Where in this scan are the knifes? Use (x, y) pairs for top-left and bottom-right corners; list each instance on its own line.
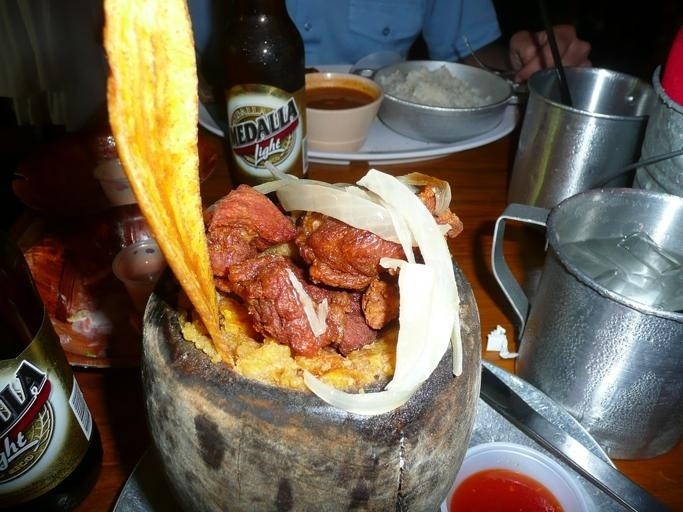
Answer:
(478, 363), (669, 512)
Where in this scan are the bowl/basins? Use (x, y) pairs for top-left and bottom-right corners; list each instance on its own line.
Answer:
(370, 60), (514, 145)
(304, 71), (385, 153)
(437, 440), (597, 512)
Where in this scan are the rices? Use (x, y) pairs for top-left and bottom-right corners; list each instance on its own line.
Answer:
(378, 65), (491, 107)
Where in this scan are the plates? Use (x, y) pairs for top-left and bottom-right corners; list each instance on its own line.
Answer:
(196, 62), (520, 168)
(113, 356), (639, 512)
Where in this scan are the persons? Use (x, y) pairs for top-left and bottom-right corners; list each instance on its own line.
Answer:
(186, 1), (594, 90)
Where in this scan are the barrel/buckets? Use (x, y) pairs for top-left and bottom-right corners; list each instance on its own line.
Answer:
(632, 63), (682, 200)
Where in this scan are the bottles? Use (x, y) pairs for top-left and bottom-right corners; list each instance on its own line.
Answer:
(218, 1), (309, 189)
(0, 227), (104, 512)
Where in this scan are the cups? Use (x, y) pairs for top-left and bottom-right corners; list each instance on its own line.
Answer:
(488, 185), (682, 463)
(504, 64), (655, 209)
